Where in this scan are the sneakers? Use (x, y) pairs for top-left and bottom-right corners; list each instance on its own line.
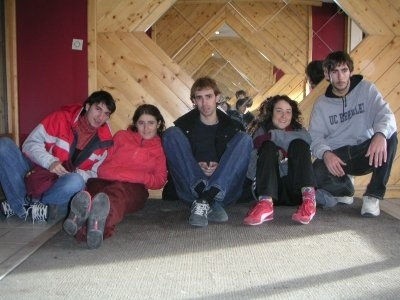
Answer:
(243, 200), (274, 225)
(361, 196), (380, 217)
(23, 197), (59, 224)
(0, 200), (17, 221)
(333, 196), (354, 205)
(189, 200), (210, 226)
(292, 192), (316, 224)
(207, 203), (228, 222)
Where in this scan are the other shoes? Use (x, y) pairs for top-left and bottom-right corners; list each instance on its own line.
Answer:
(63, 191), (91, 236)
(87, 192), (110, 249)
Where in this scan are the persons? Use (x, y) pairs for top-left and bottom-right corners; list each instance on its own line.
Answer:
(308, 51), (399, 217)
(216, 90), (274, 129)
(243, 95), (317, 225)
(306, 60), (324, 89)
(64, 104), (169, 249)
(161, 76), (254, 227)
(1, 90), (116, 225)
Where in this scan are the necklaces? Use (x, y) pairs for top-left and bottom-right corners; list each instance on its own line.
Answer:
(79, 133), (94, 149)
